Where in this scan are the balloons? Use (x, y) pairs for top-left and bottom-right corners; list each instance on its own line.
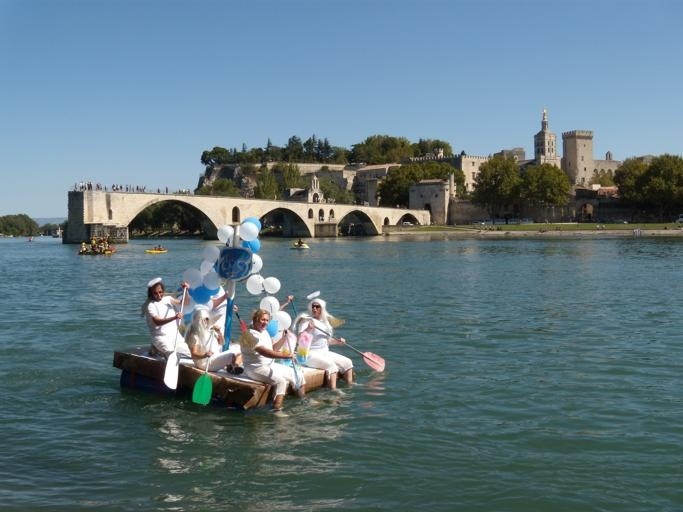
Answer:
(175, 216), (297, 353)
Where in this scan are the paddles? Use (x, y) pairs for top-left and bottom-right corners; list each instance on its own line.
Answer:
(313, 325), (385, 372)
(164, 286), (186, 390)
(235, 312), (246, 334)
(192, 330), (214, 406)
(285, 334), (303, 389)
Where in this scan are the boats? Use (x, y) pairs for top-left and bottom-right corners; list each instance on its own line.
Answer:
(26, 228), (314, 257)
(108, 331), (362, 408)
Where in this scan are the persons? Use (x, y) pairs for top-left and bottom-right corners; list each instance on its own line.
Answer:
(298, 238), (302, 245)
(80, 235), (112, 253)
(296, 298), (352, 388)
(157, 244), (161, 249)
(72, 179), (168, 194)
(181, 303), (242, 374)
(238, 310), (307, 409)
(139, 275), (192, 360)
(211, 285), (239, 355)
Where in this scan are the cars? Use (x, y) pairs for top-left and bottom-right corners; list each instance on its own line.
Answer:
(609, 217), (628, 224)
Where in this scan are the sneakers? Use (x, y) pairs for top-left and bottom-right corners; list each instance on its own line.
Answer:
(227, 366), (243, 375)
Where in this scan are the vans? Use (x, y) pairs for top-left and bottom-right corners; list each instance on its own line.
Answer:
(674, 214), (682, 223)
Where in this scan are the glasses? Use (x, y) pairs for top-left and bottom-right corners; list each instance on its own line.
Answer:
(312, 304), (320, 308)
